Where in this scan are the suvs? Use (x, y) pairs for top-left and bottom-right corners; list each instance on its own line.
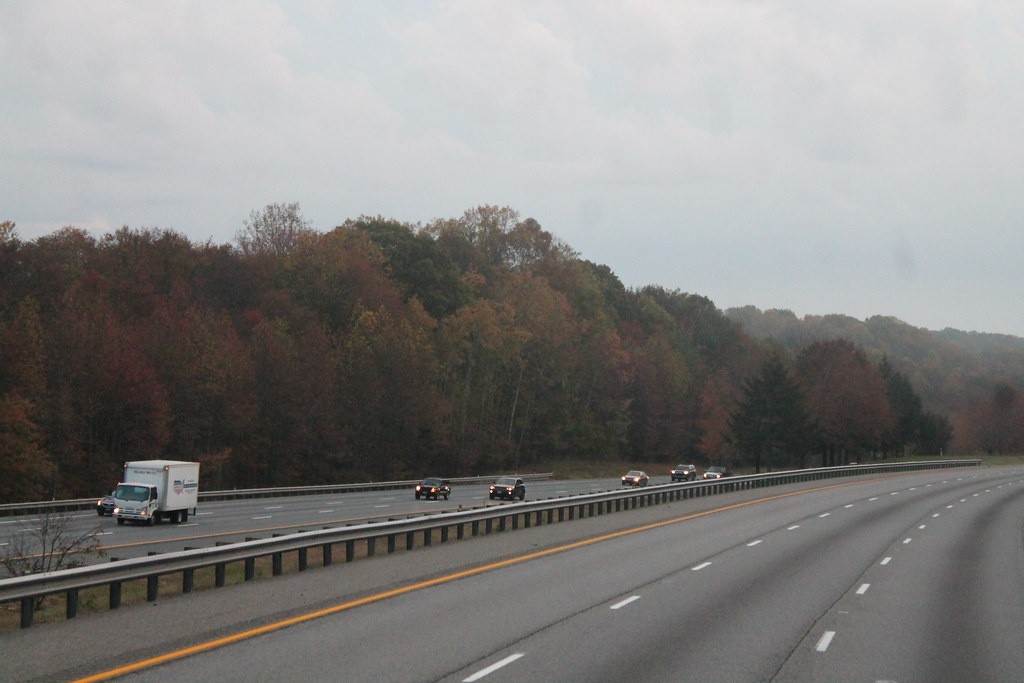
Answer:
(416, 477), (451, 501)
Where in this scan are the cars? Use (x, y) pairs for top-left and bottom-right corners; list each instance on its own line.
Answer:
(97, 494), (118, 517)
(622, 471), (649, 487)
(703, 465), (730, 479)
(490, 477), (525, 500)
(672, 464), (697, 481)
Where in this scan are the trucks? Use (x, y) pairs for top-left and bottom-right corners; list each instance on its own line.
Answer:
(116, 461), (200, 526)
